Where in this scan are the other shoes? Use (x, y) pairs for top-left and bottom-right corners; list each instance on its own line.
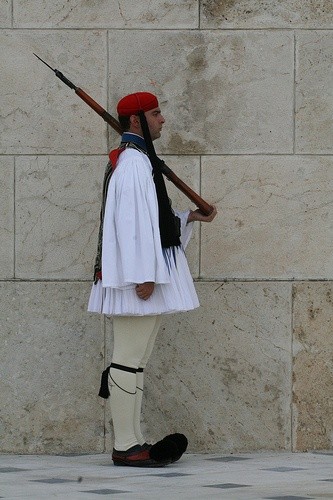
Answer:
(112, 442), (176, 467)
(142, 433), (188, 463)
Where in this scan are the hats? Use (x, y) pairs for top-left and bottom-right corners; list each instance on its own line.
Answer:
(117, 92), (158, 116)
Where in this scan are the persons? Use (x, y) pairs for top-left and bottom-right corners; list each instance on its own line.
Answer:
(87, 91), (217, 466)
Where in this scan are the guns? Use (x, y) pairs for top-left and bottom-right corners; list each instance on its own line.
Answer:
(32, 53), (214, 217)
